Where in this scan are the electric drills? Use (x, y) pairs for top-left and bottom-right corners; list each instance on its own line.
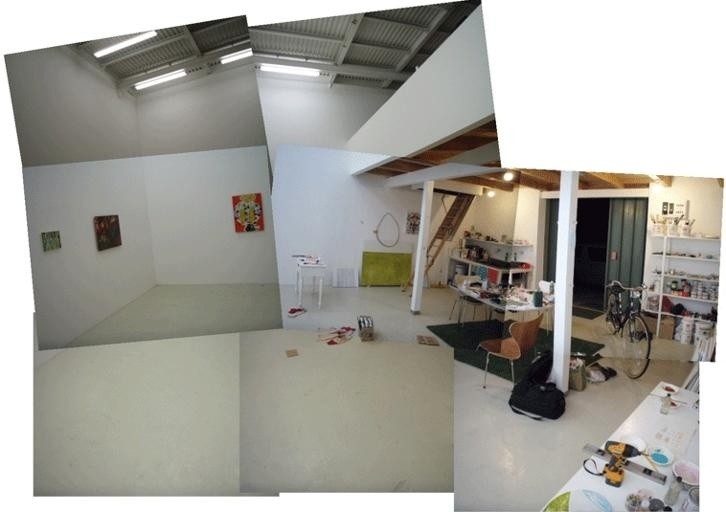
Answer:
(602, 441), (647, 473)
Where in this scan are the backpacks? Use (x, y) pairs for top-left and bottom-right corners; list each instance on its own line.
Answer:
(525, 349), (553, 386)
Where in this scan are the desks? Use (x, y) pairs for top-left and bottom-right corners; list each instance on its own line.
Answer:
(447, 281), (553, 338)
(293, 254), (326, 307)
(540, 381), (698, 512)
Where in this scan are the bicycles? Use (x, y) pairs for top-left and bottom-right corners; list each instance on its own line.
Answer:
(605, 281), (652, 380)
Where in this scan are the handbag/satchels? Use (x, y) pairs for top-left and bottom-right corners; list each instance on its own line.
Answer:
(508, 377), (567, 421)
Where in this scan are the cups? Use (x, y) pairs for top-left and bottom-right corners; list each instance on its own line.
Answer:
(483, 254), (488, 262)
(679, 486), (699, 511)
(651, 223), (695, 239)
(533, 292), (543, 307)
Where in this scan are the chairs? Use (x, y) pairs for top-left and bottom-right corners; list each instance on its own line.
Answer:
(475, 313), (544, 393)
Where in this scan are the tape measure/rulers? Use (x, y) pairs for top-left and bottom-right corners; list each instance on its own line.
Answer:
(603, 463), (625, 487)
(581, 440), (670, 486)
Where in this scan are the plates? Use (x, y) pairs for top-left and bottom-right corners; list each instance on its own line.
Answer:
(670, 399), (680, 409)
(541, 488), (618, 512)
(672, 459), (699, 487)
(620, 434), (646, 453)
(658, 382), (679, 394)
(646, 445), (673, 466)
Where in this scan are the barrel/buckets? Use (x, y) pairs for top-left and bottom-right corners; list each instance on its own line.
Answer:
(681, 318), (694, 332)
(680, 332), (693, 346)
(693, 334), (709, 347)
(694, 320), (712, 335)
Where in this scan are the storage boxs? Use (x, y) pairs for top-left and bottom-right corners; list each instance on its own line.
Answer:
(643, 312), (674, 338)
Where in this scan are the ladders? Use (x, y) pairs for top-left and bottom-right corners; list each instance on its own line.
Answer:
(402, 194), (469, 296)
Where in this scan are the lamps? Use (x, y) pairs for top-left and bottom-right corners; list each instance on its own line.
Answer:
(256, 62), (322, 77)
(219, 46), (253, 65)
(93, 30), (157, 58)
(132, 67), (188, 89)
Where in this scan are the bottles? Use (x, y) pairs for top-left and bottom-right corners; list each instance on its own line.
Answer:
(359, 317), (373, 330)
(664, 475), (682, 506)
(471, 248), (476, 257)
(663, 202), (668, 214)
(670, 279), (719, 302)
(669, 203), (674, 214)
(548, 280), (555, 294)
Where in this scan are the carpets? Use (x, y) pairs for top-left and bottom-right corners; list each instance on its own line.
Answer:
(425, 319), (605, 384)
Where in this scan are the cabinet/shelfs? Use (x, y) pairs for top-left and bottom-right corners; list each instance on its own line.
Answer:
(446, 253), (532, 296)
(644, 233), (721, 324)
